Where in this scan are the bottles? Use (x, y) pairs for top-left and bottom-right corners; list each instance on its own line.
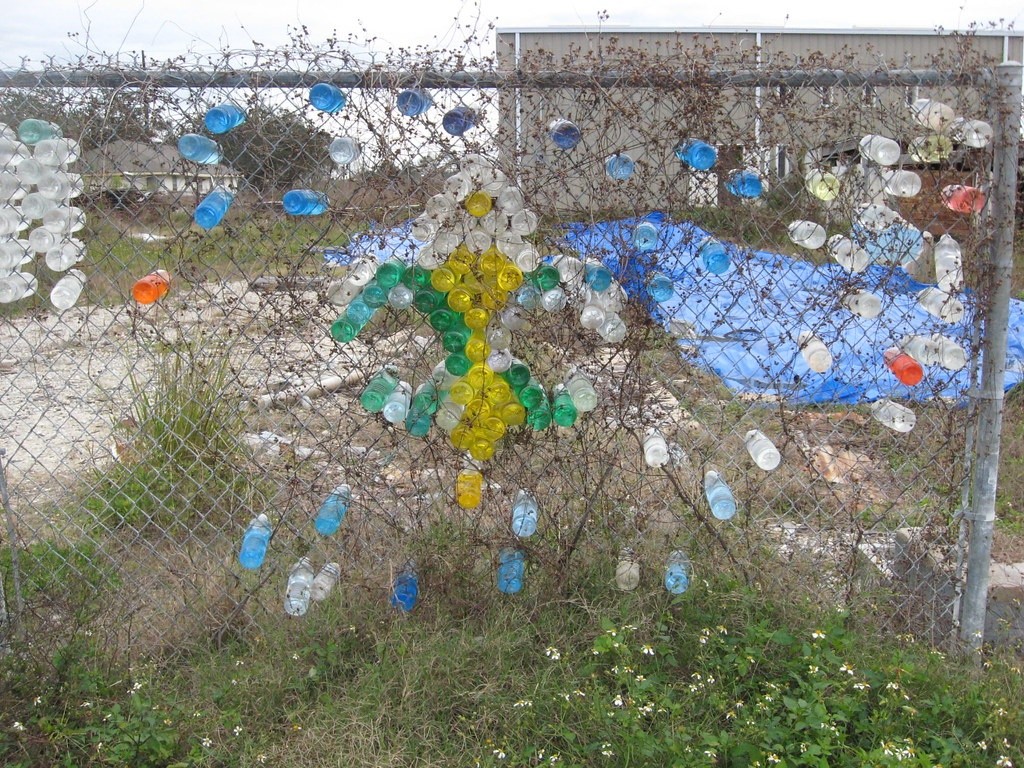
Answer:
(677, 136), (717, 171)
(238, 514), (273, 567)
(195, 184), (233, 228)
(132, 269), (170, 304)
(310, 562), (341, 600)
(664, 550), (692, 594)
(700, 235), (730, 273)
(177, 133), (226, 164)
(644, 411), (669, 469)
(617, 548), (640, 591)
(393, 556), (421, 612)
(205, 102), (247, 134)
(288, 556), (313, 615)
(646, 274), (674, 303)
(274, 83), (632, 537)
(723, 167), (763, 199)
(745, 429), (780, 471)
(499, 548), (525, 594)
(0, 119), (89, 311)
(634, 223), (660, 247)
(703, 470), (736, 520)
(787, 96), (993, 431)
